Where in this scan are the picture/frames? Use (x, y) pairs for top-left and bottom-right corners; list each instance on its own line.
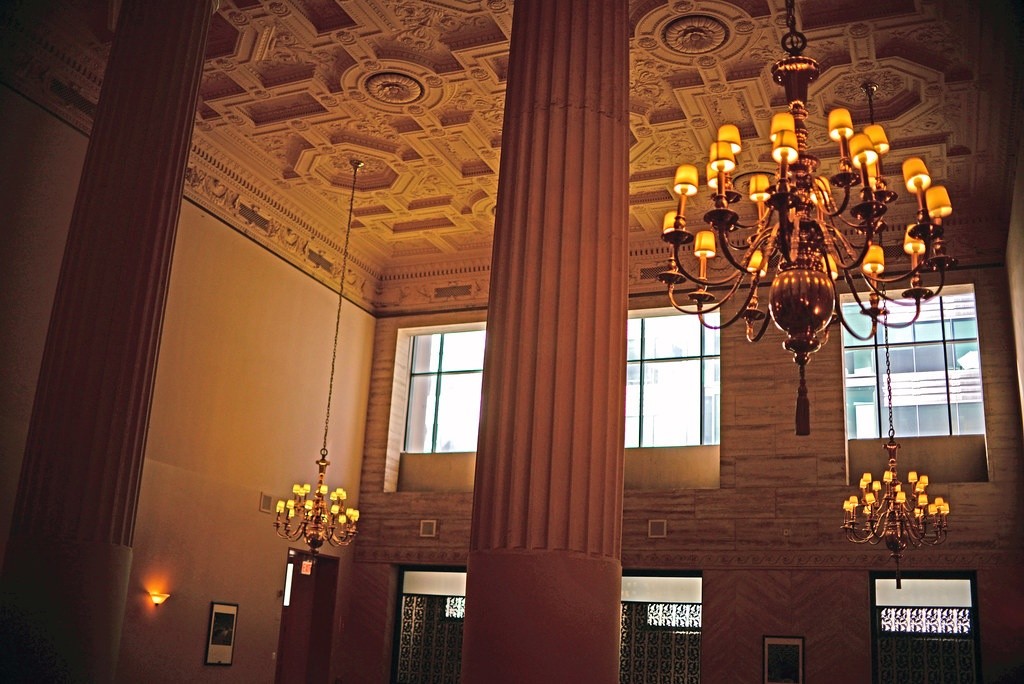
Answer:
(204, 601), (239, 669)
(761, 636), (806, 684)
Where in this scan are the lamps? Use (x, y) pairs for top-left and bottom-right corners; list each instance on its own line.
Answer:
(834, 83), (950, 596)
(148, 592), (170, 607)
(653, 0), (957, 436)
(272, 159), (365, 571)
